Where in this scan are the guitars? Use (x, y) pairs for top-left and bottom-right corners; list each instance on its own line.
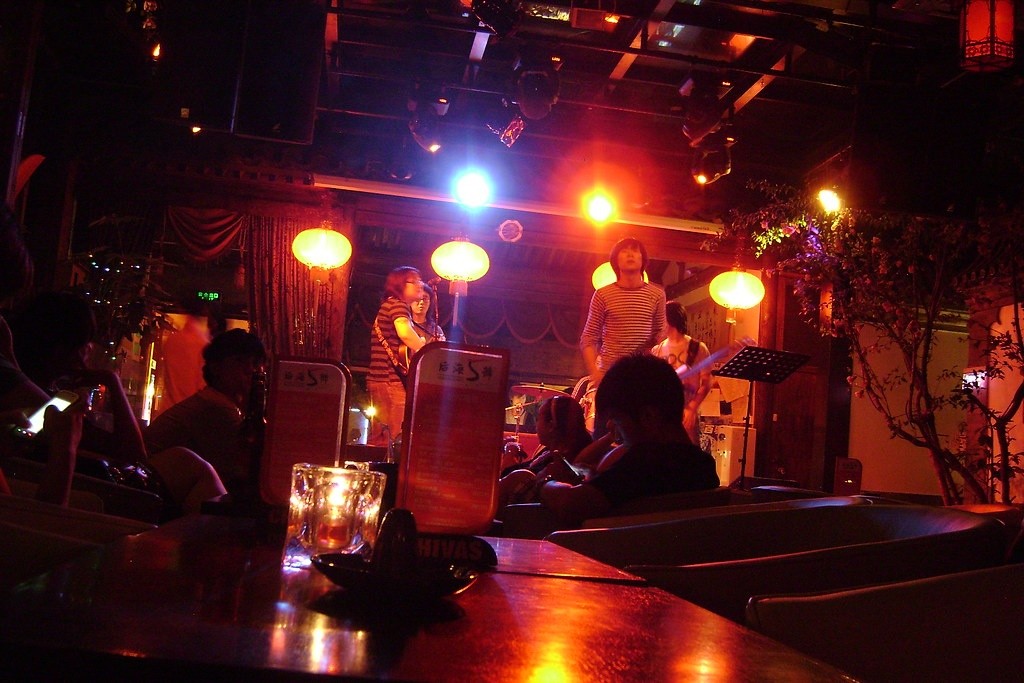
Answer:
(398, 333), (446, 376)
(678, 335), (758, 380)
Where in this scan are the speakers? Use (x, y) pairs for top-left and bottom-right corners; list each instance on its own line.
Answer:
(166, 0), (328, 145)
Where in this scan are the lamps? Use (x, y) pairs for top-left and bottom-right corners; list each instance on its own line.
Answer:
(690, 130), (736, 184)
(408, 119), (441, 151)
(434, 82), (449, 115)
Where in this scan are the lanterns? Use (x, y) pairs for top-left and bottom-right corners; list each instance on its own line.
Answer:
(591, 261), (650, 289)
(292, 219), (353, 318)
(962, 0), (1015, 76)
(708, 264), (766, 325)
(430, 231), (490, 327)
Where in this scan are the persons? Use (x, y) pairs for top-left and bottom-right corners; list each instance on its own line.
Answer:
(501, 396), (593, 512)
(543, 353), (720, 522)
(652, 302), (713, 447)
(143, 328), (262, 479)
(366, 263), (450, 449)
(578, 238), (666, 389)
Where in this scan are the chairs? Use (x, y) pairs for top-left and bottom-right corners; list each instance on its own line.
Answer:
(508, 474), (1024, 683)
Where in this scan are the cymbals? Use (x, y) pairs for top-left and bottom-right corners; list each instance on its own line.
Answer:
(511, 385), (572, 400)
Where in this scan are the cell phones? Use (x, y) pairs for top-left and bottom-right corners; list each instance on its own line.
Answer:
(14, 390), (81, 440)
(560, 456), (582, 479)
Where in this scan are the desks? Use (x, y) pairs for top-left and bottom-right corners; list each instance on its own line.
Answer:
(0, 508), (866, 683)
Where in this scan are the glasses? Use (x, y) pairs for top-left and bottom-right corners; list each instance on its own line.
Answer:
(401, 279), (423, 288)
(414, 297), (432, 304)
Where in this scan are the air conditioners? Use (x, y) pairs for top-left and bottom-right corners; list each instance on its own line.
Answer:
(486, 105), (524, 147)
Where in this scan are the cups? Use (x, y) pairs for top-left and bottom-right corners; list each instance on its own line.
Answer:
(280, 463), (385, 575)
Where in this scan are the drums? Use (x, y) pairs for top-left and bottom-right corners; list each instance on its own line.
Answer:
(579, 387), (598, 435)
